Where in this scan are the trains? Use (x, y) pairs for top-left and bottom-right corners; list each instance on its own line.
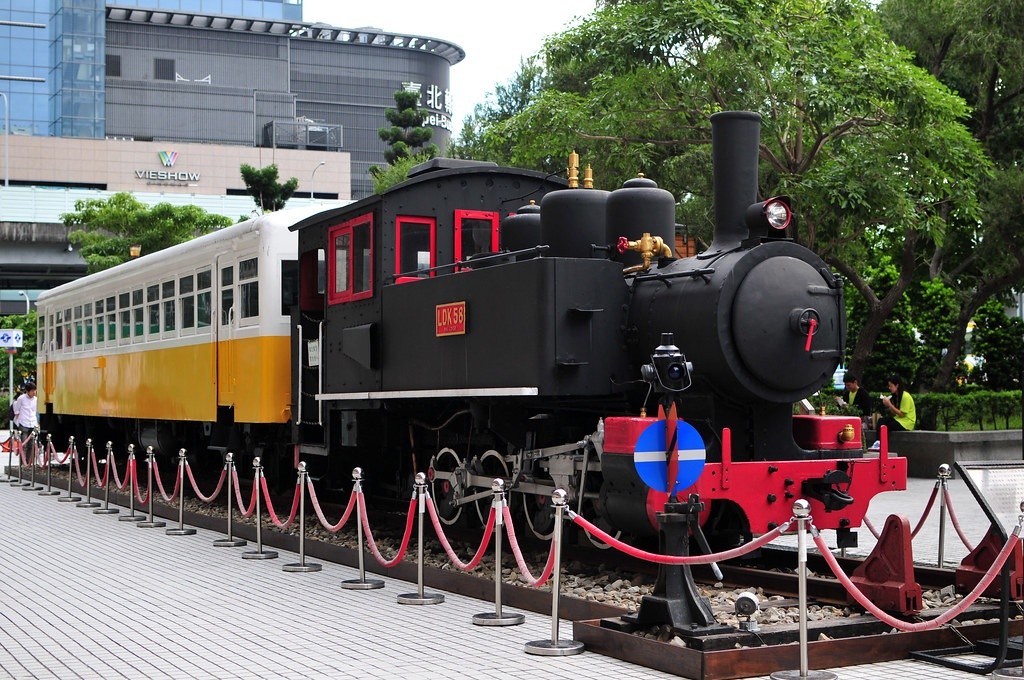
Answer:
(37, 110), (908, 570)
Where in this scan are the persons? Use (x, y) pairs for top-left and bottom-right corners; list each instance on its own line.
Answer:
(9, 392), (24, 431)
(14, 382), (37, 469)
(843, 374), (870, 416)
(868, 375), (916, 450)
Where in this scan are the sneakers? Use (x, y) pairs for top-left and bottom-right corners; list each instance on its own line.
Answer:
(868, 440), (880, 450)
